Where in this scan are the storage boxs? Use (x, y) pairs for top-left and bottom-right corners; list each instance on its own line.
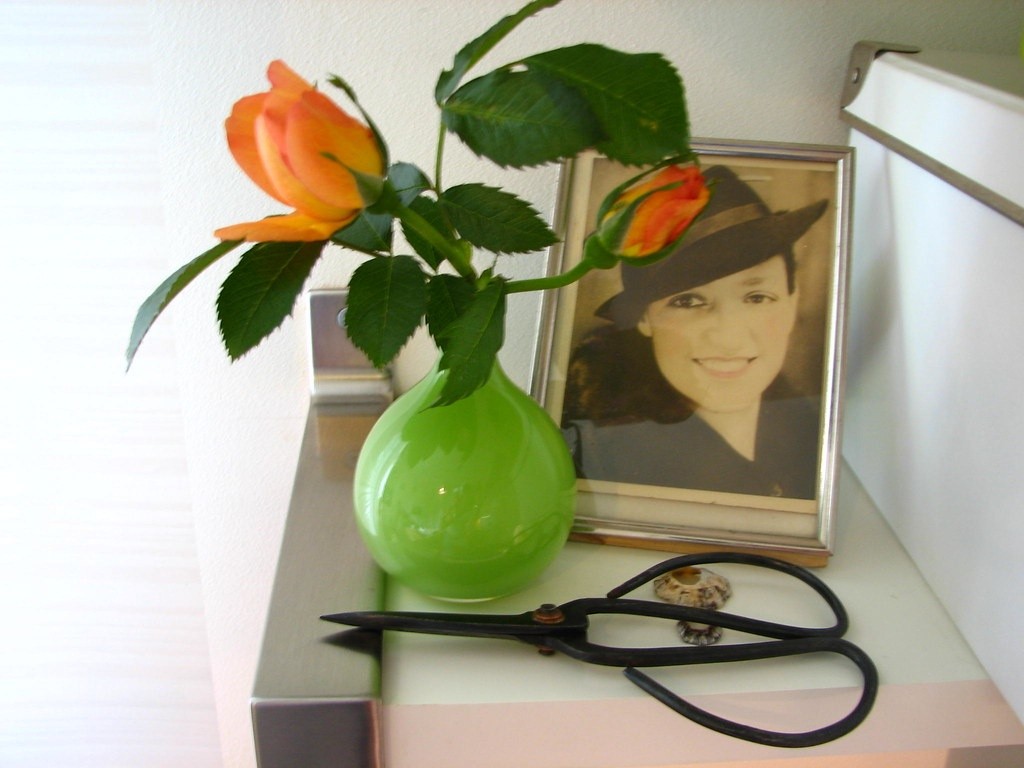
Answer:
(840, 38), (1024, 726)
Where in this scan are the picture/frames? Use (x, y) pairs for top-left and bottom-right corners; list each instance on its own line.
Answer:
(530, 137), (856, 570)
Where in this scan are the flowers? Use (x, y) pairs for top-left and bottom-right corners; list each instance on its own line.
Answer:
(124, 0), (691, 417)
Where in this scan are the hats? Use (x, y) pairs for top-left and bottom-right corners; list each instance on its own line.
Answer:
(593, 164), (829, 329)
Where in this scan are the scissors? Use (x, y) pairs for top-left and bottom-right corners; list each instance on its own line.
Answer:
(321, 550), (878, 748)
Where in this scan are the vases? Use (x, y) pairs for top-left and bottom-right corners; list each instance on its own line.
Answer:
(351, 343), (577, 603)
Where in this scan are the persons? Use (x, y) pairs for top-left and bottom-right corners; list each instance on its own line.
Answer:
(560, 167), (829, 501)
